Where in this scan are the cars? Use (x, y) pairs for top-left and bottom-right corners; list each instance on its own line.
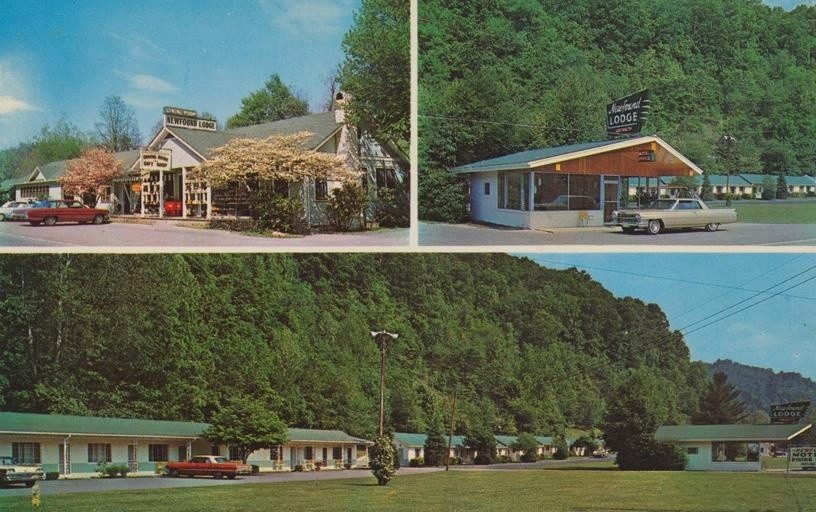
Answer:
(534, 191), (613, 211)
(613, 197), (739, 233)
(592, 447), (610, 458)
(0, 455), (46, 489)
(165, 454), (255, 480)
(0, 200), (40, 222)
(11, 198), (111, 226)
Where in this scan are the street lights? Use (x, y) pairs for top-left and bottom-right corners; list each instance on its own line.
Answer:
(371, 328), (401, 440)
